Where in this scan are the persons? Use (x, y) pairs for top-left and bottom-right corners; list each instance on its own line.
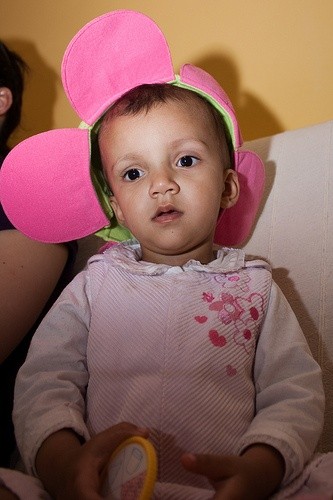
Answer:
(0, 39), (78, 467)
(0, 8), (333, 499)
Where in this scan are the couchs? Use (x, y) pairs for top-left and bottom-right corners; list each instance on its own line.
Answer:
(66, 120), (333, 454)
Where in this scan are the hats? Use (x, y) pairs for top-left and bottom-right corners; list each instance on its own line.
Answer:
(1, 10), (266, 254)
